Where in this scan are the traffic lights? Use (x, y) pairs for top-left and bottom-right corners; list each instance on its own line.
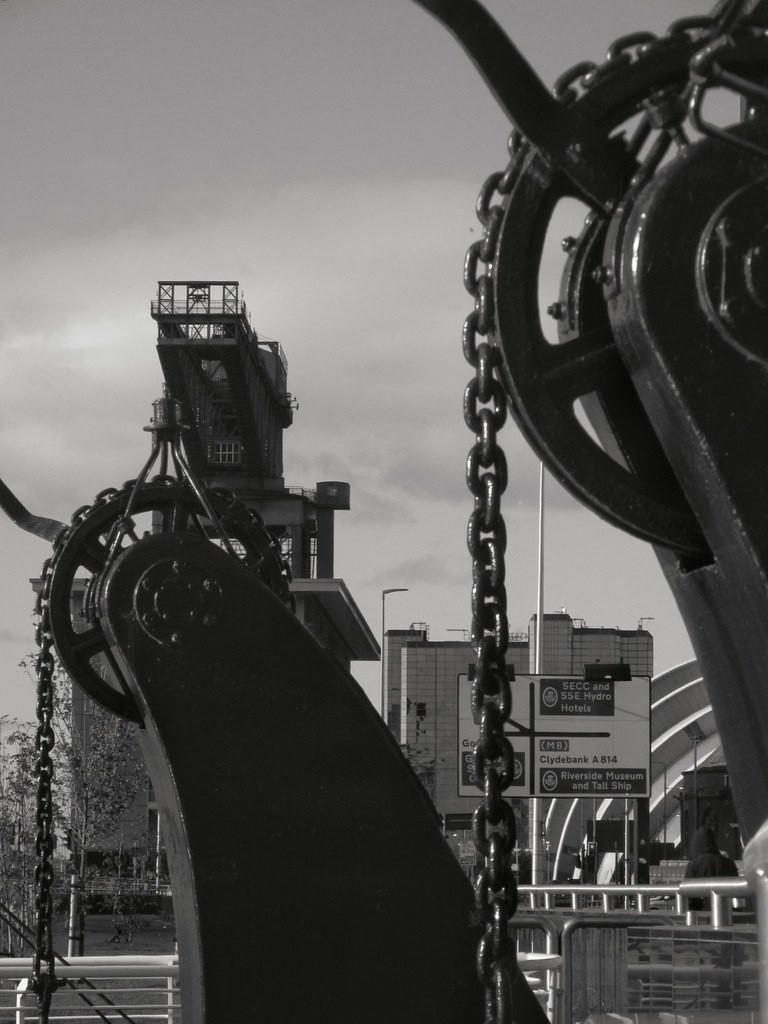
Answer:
(380, 589), (410, 720)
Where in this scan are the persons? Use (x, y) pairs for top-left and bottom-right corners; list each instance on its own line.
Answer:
(685, 826), (738, 911)
